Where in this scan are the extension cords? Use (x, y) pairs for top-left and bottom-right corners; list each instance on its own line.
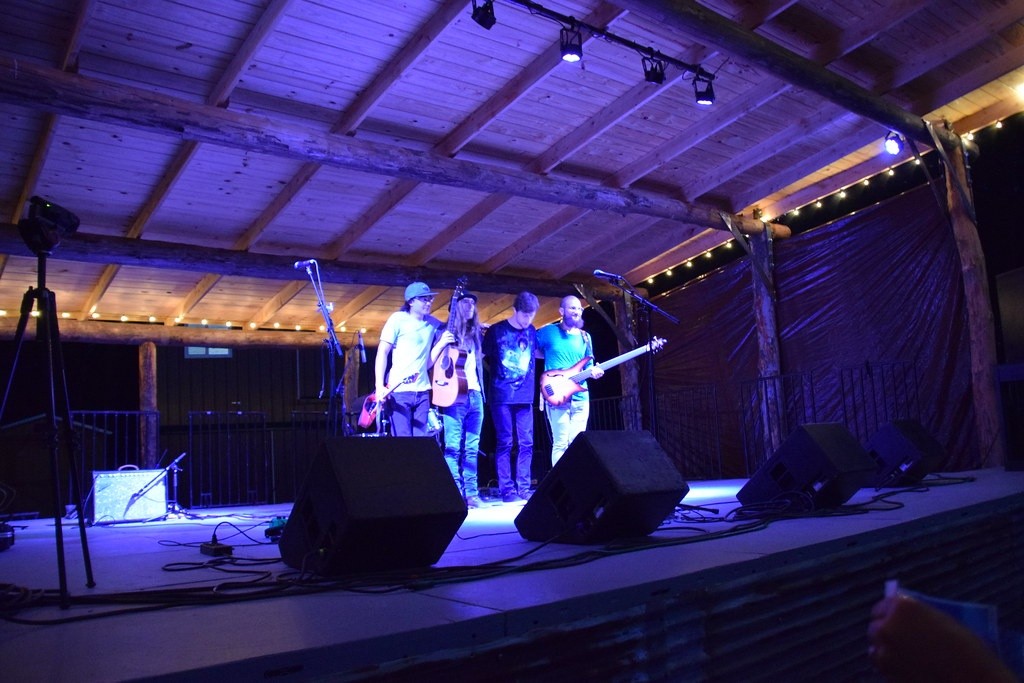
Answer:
(201, 542), (235, 557)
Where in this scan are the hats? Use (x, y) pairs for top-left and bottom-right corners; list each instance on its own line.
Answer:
(447, 290), (477, 313)
(404, 281), (438, 302)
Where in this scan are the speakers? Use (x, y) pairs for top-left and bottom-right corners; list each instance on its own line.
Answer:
(736, 423), (876, 519)
(91, 469), (168, 524)
(515, 429), (691, 544)
(863, 418), (945, 489)
(279, 435), (468, 578)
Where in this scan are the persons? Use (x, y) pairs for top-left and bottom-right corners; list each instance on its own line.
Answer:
(483, 295), (605, 469)
(426, 290), (487, 509)
(867, 595), (1022, 683)
(374, 282), (490, 437)
(481, 291), (545, 503)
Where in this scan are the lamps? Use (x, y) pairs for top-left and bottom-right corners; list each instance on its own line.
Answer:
(692, 68), (715, 105)
(471, 0), (496, 30)
(885, 131), (903, 155)
(641, 47), (666, 84)
(560, 16), (583, 62)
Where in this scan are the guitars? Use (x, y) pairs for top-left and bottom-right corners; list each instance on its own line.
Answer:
(427, 275), (468, 408)
(540, 336), (667, 406)
(358, 372), (420, 429)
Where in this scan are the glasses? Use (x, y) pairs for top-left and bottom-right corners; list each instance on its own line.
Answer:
(414, 296), (435, 303)
(563, 306), (585, 312)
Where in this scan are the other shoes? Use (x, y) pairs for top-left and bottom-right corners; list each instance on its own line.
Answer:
(465, 496), (491, 510)
(502, 489), (524, 505)
(519, 488), (533, 500)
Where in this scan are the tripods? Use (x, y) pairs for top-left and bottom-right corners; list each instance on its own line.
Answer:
(138, 454), (204, 522)
(0, 253), (98, 609)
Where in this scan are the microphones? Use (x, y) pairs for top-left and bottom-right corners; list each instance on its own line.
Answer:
(132, 492), (139, 497)
(593, 269), (624, 280)
(358, 332), (366, 362)
(294, 259), (315, 269)
